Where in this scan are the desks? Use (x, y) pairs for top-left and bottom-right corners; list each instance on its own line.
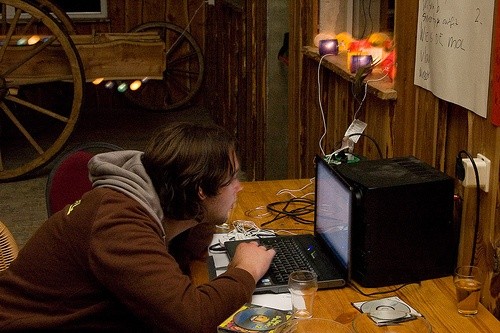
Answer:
(187, 177), (500, 333)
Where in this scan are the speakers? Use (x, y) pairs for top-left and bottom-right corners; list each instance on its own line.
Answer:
(350, 55), (372, 74)
(332, 155), (458, 288)
(319, 39), (339, 56)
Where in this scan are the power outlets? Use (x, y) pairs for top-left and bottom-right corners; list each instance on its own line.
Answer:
(476, 152), (491, 193)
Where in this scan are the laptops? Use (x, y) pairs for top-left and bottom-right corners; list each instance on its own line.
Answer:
(223, 154), (356, 294)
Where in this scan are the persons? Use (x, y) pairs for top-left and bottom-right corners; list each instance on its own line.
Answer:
(0, 119), (276, 333)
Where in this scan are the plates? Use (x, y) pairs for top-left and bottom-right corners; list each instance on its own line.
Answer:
(352, 310), (431, 333)
(269, 318), (352, 333)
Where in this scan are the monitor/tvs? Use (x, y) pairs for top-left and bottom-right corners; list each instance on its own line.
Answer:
(6, 0), (108, 19)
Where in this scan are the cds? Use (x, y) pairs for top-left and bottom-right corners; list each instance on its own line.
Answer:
(361, 299), (411, 320)
(233, 306), (287, 331)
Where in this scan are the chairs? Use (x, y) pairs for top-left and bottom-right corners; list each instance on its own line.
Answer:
(44, 140), (128, 218)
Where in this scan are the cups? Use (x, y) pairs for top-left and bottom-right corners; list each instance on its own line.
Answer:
(453, 265), (483, 315)
(287, 271), (317, 320)
